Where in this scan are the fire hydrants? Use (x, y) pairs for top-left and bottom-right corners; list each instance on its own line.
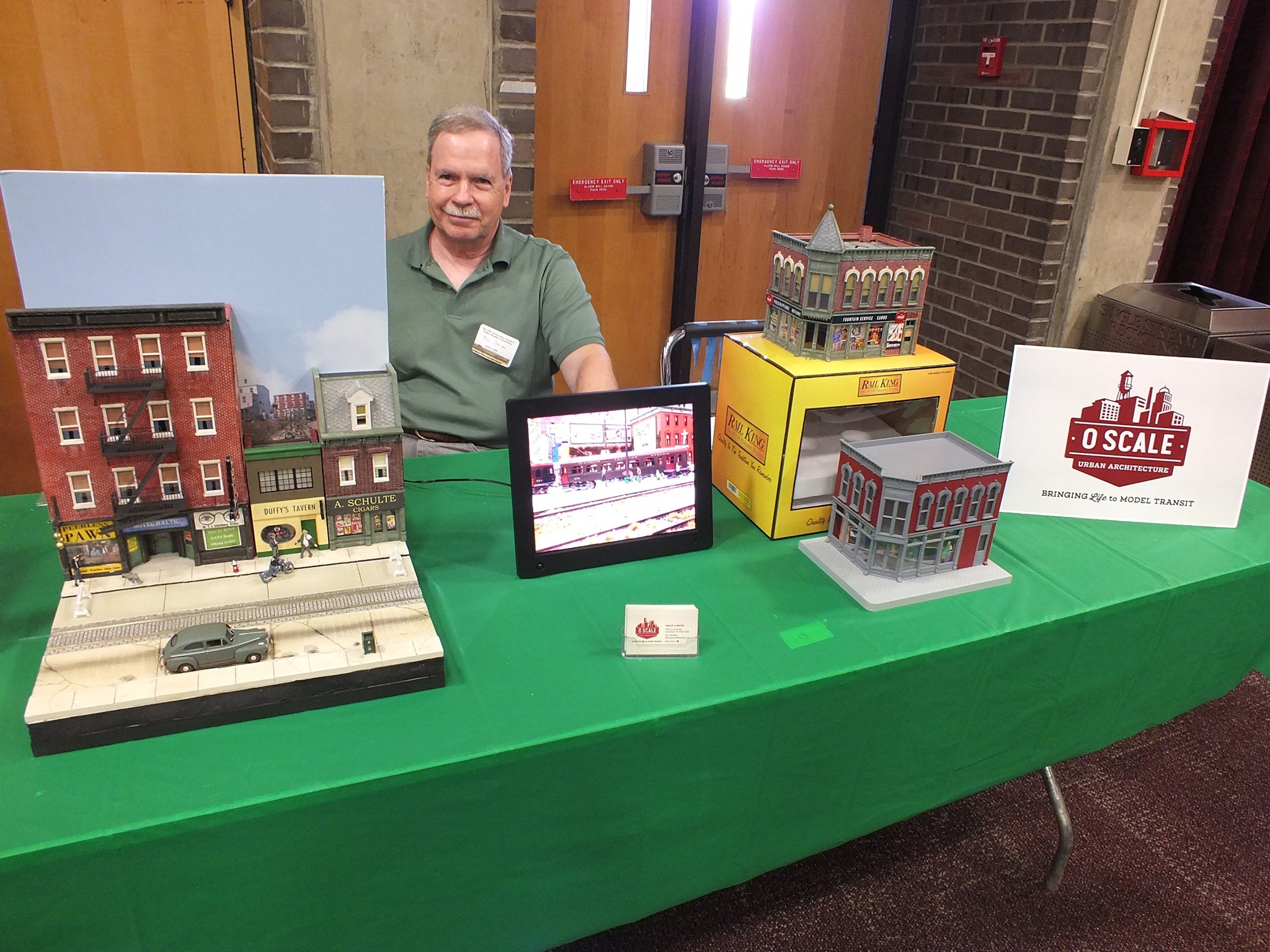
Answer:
(230, 560), (239, 572)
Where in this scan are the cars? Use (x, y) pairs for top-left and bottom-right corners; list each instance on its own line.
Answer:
(158, 620), (272, 674)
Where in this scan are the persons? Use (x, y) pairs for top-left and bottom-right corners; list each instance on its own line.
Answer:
(269, 554), (280, 577)
(295, 530), (319, 559)
(270, 532), (279, 558)
(71, 556), (84, 587)
(385, 105), (619, 456)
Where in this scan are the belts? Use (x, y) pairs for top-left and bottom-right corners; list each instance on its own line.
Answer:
(402, 426), (467, 444)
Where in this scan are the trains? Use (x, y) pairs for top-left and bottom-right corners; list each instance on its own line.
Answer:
(530, 446), (691, 494)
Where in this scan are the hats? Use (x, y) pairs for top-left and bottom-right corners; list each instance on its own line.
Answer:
(263, 522), (321, 543)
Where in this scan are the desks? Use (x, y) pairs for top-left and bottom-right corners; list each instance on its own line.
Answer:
(0, 394), (1270, 952)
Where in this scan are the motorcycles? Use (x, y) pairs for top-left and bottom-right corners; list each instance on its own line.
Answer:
(258, 558), (296, 583)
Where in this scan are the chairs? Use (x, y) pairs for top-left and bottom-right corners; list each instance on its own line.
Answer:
(659, 318), (766, 452)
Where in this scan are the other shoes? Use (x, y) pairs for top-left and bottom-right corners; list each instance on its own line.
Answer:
(271, 572), (278, 578)
(299, 552), (314, 559)
(75, 580), (85, 587)
